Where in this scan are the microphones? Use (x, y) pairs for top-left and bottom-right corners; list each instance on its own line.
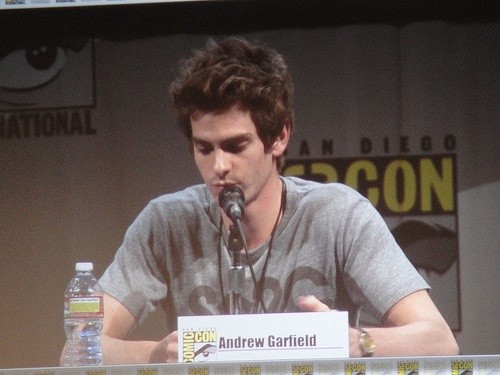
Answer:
(218, 186), (245, 222)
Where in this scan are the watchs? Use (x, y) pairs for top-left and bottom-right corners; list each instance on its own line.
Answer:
(354, 325), (376, 359)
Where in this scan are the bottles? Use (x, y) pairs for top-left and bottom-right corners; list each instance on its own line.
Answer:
(59, 262), (104, 365)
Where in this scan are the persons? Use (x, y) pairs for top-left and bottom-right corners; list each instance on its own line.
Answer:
(57, 35), (460, 370)
